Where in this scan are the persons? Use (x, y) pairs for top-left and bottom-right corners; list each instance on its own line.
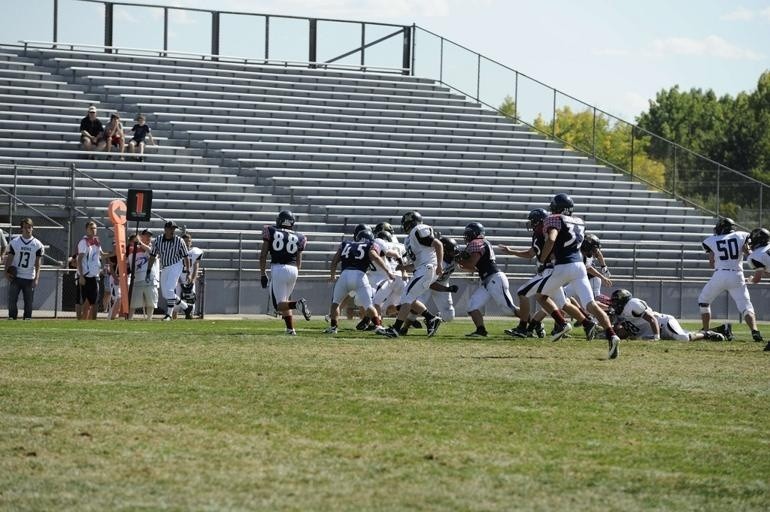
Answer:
(258, 209), (312, 336)
(123, 114), (159, 164)
(68, 219), (205, 324)
(78, 105), (108, 163)
(102, 114), (127, 161)
(0, 229), (8, 266)
(5, 217), (45, 320)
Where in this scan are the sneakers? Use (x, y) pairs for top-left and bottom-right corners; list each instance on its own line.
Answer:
(295, 297), (312, 321)
(354, 316), (382, 332)
(610, 288), (633, 316)
(550, 320), (572, 342)
(607, 333), (621, 359)
(285, 327), (297, 336)
(751, 330), (763, 341)
(422, 312), (443, 338)
(84, 155), (145, 162)
(161, 313), (173, 321)
(503, 319), (547, 339)
(704, 323), (734, 342)
(573, 314), (598, 342)
(374, 318), (422, 338)
(183, 303), (194, 317)
(464, 326), (490, 338)
(321, 315), (338, 335)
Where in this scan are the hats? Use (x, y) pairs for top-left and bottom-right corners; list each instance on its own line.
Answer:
(164, 220), (179, 229)
(141, 228), (153, 236)
(88, 106), (97, 113)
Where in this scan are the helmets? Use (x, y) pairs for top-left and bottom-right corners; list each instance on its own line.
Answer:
(462, 222), (486, 244)
(400, 209), (425, 235)
(746, 227), (770, 249)
(580, 232), (601, 259)
(275, 210), (296, 229)
(439, 236), (461, 261)
(712, 217), (737, 236)
(547, 192), (575, 216)
(352, 221), (395, 242)
(526, 207), (549, 231)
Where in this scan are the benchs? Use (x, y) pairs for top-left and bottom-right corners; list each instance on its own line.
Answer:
(0, 39), (769, 293)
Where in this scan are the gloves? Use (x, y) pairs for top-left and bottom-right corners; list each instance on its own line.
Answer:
(536, 259), (545, 269)
(260, 272), (269, 288)
(649, 333), (661, 342)
(747, 274), (754, 283)
(601, 264), (612, 280)
(447, 284), (459, 293)
